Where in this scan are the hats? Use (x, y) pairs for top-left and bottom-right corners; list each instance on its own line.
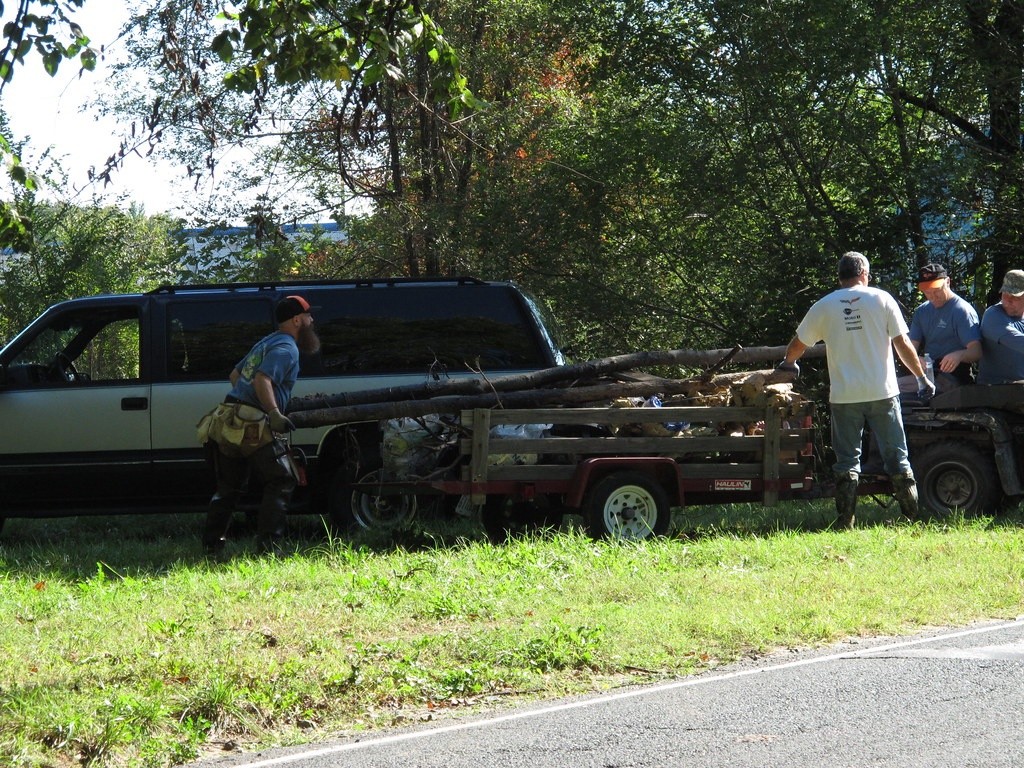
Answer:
(910, 264), (948, 283)
(276, 295), (323, 322)
(999, 269), (1024, 296)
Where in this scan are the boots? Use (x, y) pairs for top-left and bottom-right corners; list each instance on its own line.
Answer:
(889, 469), (919, 522)
(835, 471), (859, 530)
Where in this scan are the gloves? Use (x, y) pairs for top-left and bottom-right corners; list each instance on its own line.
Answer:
(779, 356), (801, 379)
(267, 408), (295, 434)
(917, 375), (937, 401)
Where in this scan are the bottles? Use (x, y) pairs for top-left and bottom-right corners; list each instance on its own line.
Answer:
(922, 352), (935, 384)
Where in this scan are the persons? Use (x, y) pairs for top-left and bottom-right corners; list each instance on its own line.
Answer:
(775, 251), (936, 532)
(908, 264), (983, 389)
(202, 295), (321, 562)
(978, 269), (1024, 387)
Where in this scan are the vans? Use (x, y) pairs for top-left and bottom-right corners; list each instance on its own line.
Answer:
(0, 276), (564, 545)
